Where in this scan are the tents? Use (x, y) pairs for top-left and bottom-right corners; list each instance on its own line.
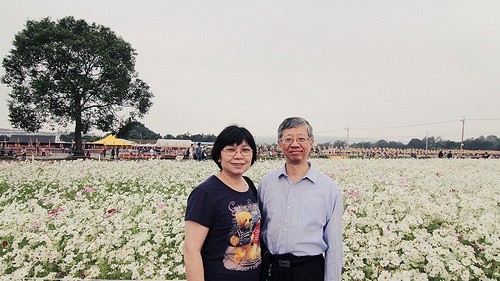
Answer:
(92, 134), (133, 149)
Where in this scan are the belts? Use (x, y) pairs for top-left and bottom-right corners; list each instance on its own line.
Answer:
(274, 257), (312, 268)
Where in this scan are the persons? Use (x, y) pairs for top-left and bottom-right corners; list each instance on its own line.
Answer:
(257, 117), (343, 281)
(268, 149), (284, 158)
(438, 151), (443, 158)
(183, 124), (263, 281)
(8, 148), (14, 156)
(410, 152), (416, 158)
(129, 148), (132, 156)
(189, 142), (208, 161)
(86, 151), (91, 157)
(41, 147), (46, 157)
(35, 147), (40, 156)
(0, 147), (6, 157)
(150, 147), (161, 159)
(482, 152), (489, 158)
(447, 151), (452, 158)
(111, 148), (119, 160)
(100, 147), (106, 158)
(21, 147), (27, 161)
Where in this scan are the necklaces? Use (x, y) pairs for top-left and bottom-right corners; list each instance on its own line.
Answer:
(219, 170), (247, 191)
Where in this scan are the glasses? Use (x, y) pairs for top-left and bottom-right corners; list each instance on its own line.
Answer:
(281, 135), (310, 142)
(222, 148), (253, 155)
